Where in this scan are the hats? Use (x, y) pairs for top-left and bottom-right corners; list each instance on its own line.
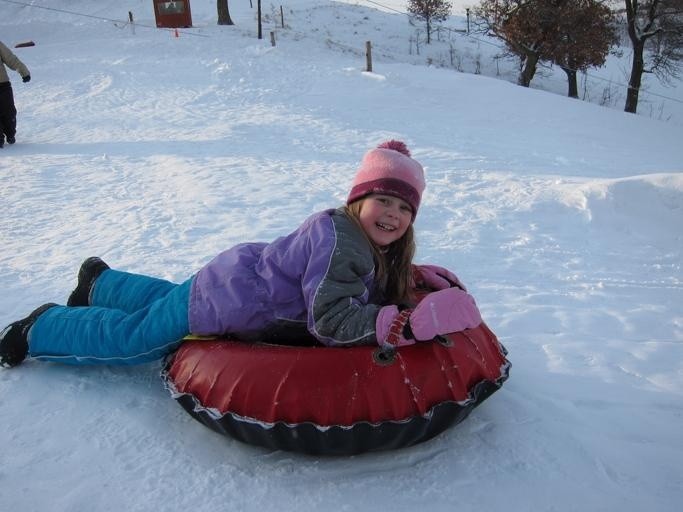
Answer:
(346, 140), (426, 225)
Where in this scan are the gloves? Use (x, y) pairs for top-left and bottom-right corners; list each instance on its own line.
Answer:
(22, 75), (31, 82)
(375, 286), (483, 348)
(409, 264), (467, 290)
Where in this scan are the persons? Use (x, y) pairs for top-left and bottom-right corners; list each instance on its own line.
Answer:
(1, 139), (483, 367)
(0, 40), (31, 150)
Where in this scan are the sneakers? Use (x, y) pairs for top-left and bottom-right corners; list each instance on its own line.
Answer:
(66, 256), (111, 307)
(0, 303), (58, 369)
(0, 135), (15, 148)
